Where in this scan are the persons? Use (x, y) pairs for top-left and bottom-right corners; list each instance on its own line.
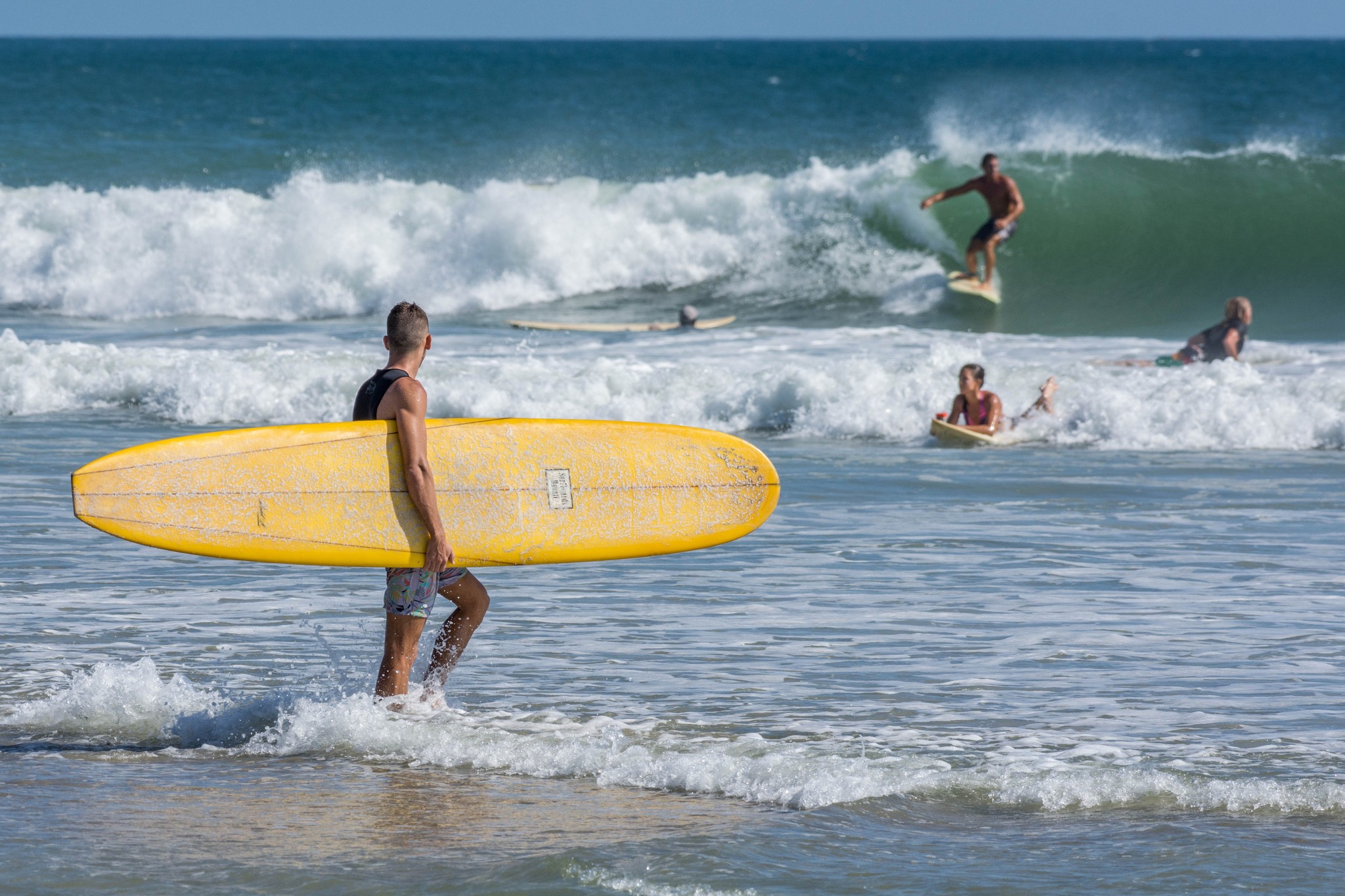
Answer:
(920, 153), (1024, 290)
(1094, 296), (1252, 367)
(351, 301), (489, 715)
(650, 304), (704, 333)
(931, 364), (1058, 436)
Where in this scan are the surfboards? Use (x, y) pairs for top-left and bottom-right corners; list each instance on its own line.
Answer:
(930, 418), (993, 445)
(71, 418), (781, 569)
(945, 268), (1002, 305)
(506, 314), (735, 332)
